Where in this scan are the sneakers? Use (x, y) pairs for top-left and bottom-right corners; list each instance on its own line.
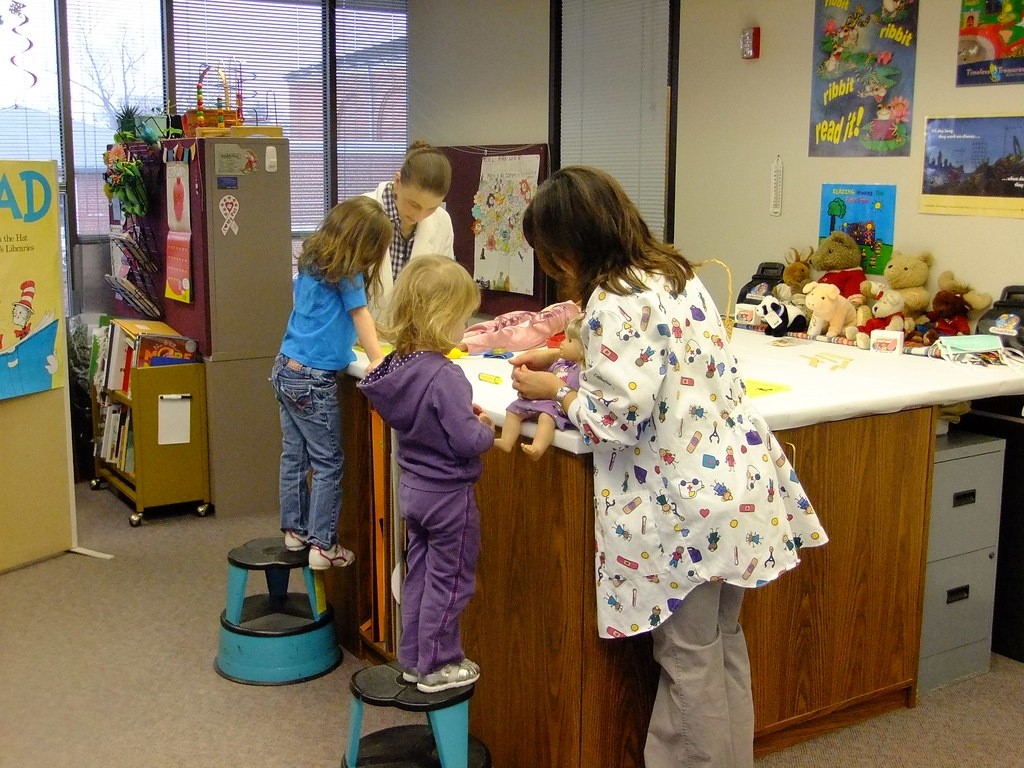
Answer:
(308, 542), (355, 573)
(284, 530), (312, 551)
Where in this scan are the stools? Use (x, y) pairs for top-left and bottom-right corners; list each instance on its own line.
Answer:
(340, 659), (492, 768)
(213, 535), (345, 686)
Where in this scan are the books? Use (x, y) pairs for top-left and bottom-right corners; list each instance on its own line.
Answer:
(94, 230), (193, 474)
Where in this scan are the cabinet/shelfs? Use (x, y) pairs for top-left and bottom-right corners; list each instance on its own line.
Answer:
(90, 317), (210, 526)
(106, 138), (292, 519)
(306, 325), (1024, 768)
(917, 431), (1006, 695)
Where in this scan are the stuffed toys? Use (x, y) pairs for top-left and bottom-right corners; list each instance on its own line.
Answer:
(756, 228), (992, 349)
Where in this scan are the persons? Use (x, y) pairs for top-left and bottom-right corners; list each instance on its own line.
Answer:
(454, 297), (588, 460)
(516, 166), (830, 768)
(357, 142), (455, 340)
(272, 194), (395, 569)
(359, 253), (496, 689)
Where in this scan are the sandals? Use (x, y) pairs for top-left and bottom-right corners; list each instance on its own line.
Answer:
(402, 657), (480, 693)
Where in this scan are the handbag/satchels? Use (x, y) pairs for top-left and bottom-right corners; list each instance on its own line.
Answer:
(736, 262), (786, 307)
(975, 285), (1024, 356)
